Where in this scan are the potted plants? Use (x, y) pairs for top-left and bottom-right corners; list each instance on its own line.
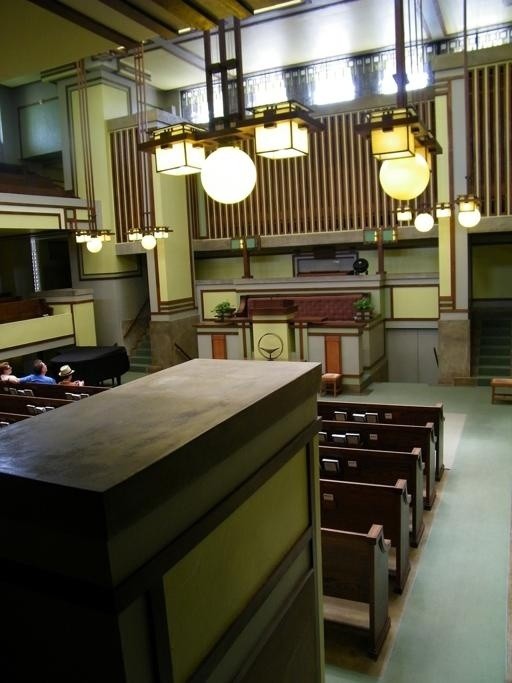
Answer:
(211, 301), (236, 322)
(353, 297), (376, 323)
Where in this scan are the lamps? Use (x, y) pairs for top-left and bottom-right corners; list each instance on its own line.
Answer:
(453, 1), (482, 228)
(124, 41), (174, 250)
(389, 0), (455, 233)
(356, 1), (443, 201)
(137, 18), (326, 204)
(72, 57), (115, 253)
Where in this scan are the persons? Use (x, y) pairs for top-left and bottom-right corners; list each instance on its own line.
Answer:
(8, 360), (57, 384)
(0, 362), (19, 383)
(58, 365), (84, 385)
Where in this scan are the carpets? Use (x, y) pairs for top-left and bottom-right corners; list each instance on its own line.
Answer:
(103, 370), (511, 682)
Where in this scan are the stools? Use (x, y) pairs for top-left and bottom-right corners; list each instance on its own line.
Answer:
(320, 373), (343, 398)
(491, 379), (512, 404)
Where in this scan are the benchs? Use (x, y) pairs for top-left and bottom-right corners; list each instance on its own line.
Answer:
(0, 394), (74, 414)
(1, 412), (33, 423)
(318, 401), (444, 482)
(0, 380), (110, 399)
(319, 445), (425, 549)
(0, 421), (9, 428)
(320, 420), (437, 511)
(321, 525), (391, 662)
(319, 478), (411, 595)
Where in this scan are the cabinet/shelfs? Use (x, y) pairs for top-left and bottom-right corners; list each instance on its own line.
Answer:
(0, 358), (324, 682)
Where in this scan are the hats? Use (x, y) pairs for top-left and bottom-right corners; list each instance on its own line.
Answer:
(58, 365), (75, 377)
